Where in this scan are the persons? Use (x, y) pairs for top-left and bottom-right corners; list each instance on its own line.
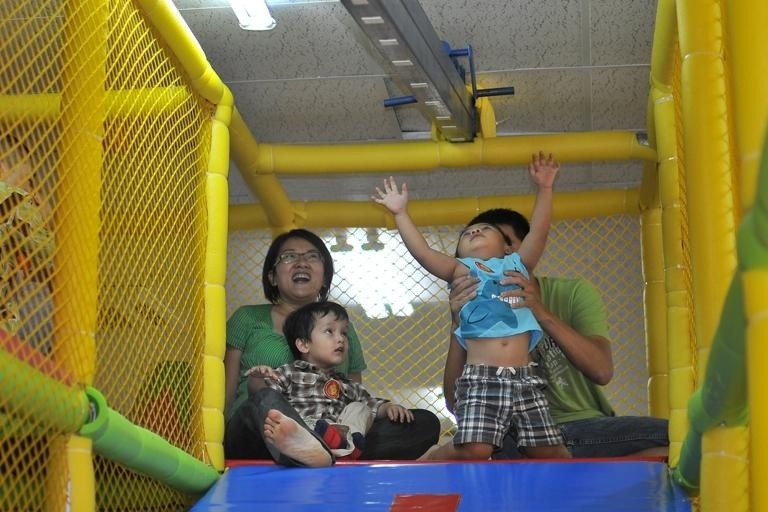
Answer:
(370, 151), (573, 458)
(444, 208), (669, 458)
(226, 229), (441, 469)
(243, 301), (415, 462)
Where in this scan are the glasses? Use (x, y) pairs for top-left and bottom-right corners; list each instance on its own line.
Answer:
(271, 252), (324, 270)
